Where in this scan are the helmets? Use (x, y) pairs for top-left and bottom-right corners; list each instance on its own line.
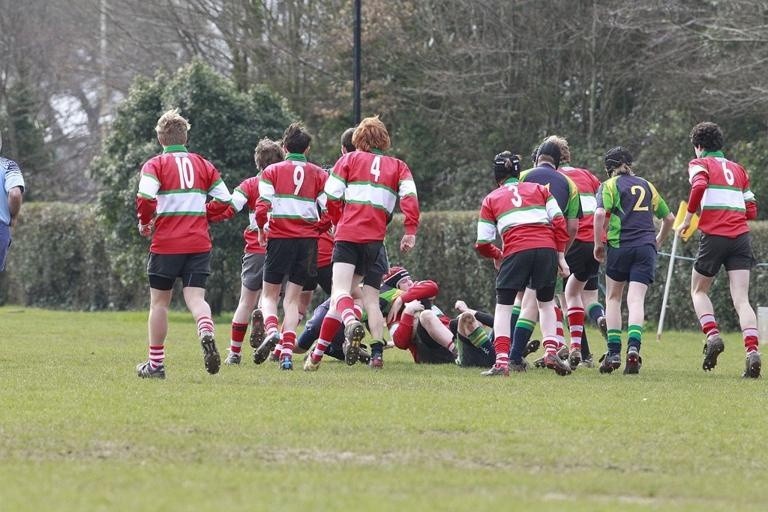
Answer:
(381, 266), (411, 290)
(493, 151), (520, 181)
(535, 141), (562, 167)
(605, 146), (633, 172)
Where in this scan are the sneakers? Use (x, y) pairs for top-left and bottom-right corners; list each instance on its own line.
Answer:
(136, 361), (166, 381)
(225, 309), (383, 372)
(742, 352), (763, 379)
(482, 316), (641, 377)
(701, 335), (725, 371)
(199, 331), (221, 375)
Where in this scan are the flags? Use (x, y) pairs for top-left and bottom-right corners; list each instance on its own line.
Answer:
(671, 199), (702, 245)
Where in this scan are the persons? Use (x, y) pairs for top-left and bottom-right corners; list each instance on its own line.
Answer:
(134, 104), (231, 378)
(677, 120), (762, 380)
(0, 138), (27, 286)
(591, 147), (677, 374)
(475, 135), (606, 376)
(277, 266), (540, 367)
(211, 116), (419, 372)
